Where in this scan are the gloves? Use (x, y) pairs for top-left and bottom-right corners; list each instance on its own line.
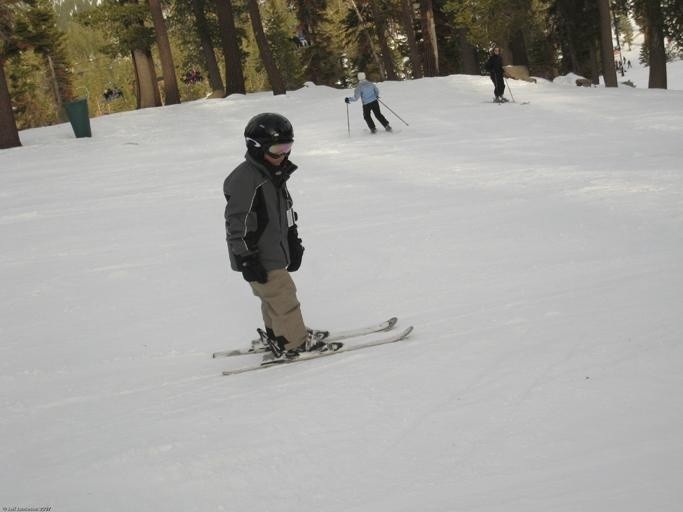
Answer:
(287, 241), (302, 271)
(240, 255), (267, 284)
(345, 97), (348, 103)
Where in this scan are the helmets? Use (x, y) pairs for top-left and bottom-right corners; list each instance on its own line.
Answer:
(357, 72), (366, 80)
(244, 112), (294, 169)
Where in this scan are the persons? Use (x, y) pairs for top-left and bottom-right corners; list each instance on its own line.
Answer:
(345, 71), (393, 133)
(222, 111), (329, 360)
(483, 47), (510, 103)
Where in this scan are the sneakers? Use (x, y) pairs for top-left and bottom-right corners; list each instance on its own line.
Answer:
(386, 125), (390, 130)
(371, 127), (376, 132)
(494, 96), (507, 103)
(285, 327), (327, 352)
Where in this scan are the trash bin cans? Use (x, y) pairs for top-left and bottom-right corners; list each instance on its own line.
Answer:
(63, 98), (91, 138)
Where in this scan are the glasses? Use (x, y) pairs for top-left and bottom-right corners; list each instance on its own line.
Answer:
(245, 136), (293, 158)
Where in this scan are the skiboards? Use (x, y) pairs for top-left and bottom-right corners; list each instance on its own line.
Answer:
(482, 100), (530, 105)
(363, 129), (402, 135)
(212, 316), (413, 377)
